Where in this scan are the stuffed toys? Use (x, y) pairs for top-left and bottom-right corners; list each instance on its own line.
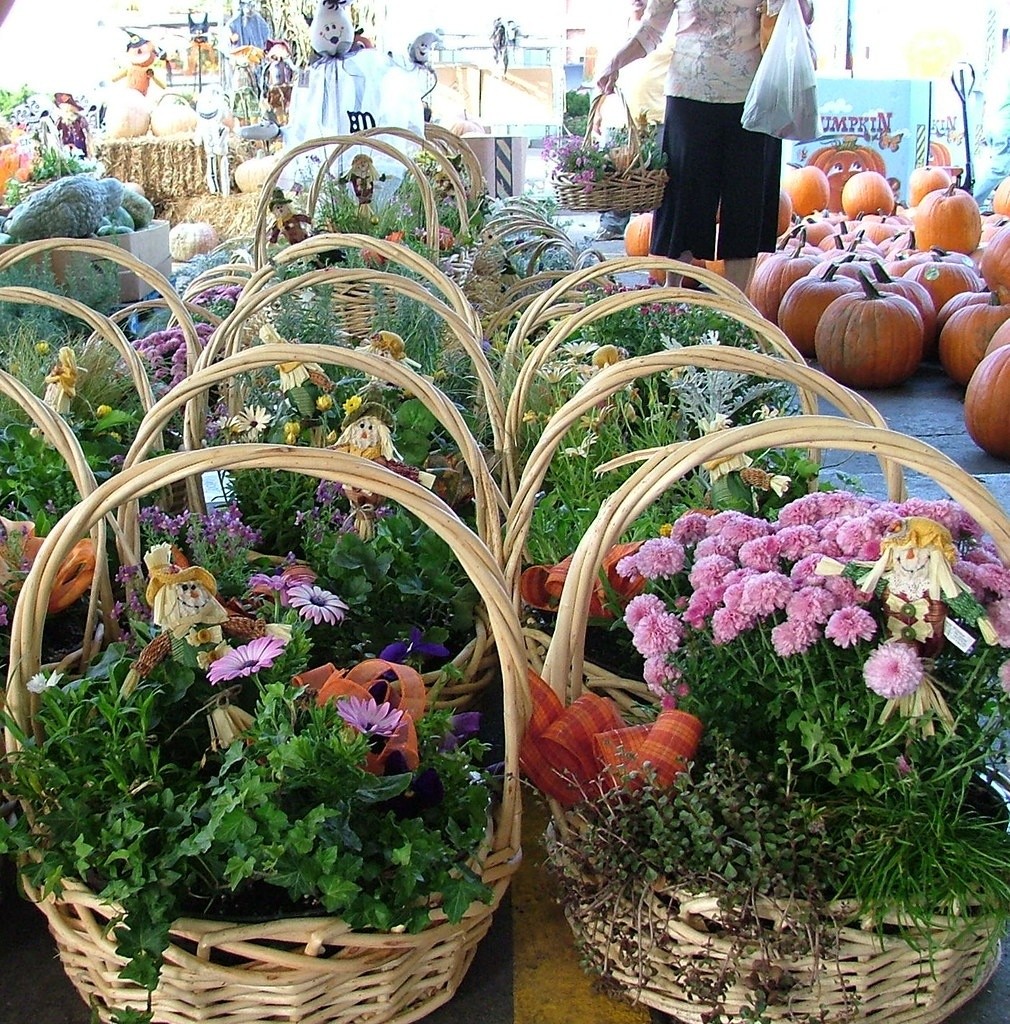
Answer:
(194, 82), (232, 197)
(308, 0), (355, 58)
(409, 32), (442, 66)
(111, 27), (167, 96)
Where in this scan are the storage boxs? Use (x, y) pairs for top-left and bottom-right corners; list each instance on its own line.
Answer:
(1, 220), (172, 302)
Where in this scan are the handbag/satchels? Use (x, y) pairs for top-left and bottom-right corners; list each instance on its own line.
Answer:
(738, 0), (825, 147)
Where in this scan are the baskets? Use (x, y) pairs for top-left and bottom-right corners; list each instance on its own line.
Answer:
(551, 84), (667, 215)
(8, 443), (532, 1020)
(0, 123), (908, 732)
(543, 416), (1000, 1024)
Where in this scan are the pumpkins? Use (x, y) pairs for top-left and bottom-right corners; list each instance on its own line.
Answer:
(0, 89), (281, 263)
(622, 165), (1010, 463)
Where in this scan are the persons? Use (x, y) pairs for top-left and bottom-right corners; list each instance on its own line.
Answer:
(8, 86), (92, 169)
(697, 413), (791, 518)
(597, 0), (818, 302)
(338, 153), (388, 224)
(265, 186), (313, 251)
(815, 517), (1000, 739)
(325, 403), (436, 544)
(118, 545), (292, 752)
(230, 38), (298, 93)
(43, 347), (77, 414)
(355, 330), (406, 396)
